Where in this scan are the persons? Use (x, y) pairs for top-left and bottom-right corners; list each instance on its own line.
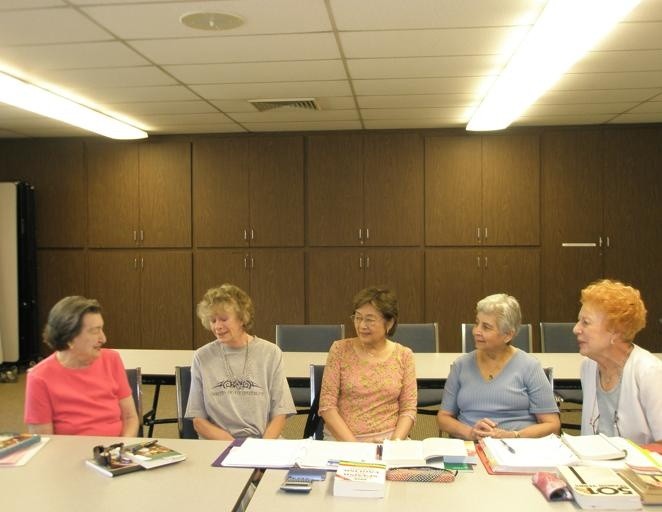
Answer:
(183, 285), (297, 441)
(572, 279), (662, 457)
(317, 288), (419, 442)
(436, 292), (561, 442)
(24, 296), (141, 438)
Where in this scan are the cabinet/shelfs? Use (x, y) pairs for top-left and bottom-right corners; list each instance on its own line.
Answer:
(193, 132), (304, 248)
(305, 248), (425, 325)
(542, 130), (660, 246)
(544, 246), (661, 354)
(0, 138), (85, 249)
(425, 132), (543, 248)
(193, 248), (305, 350)
(85, 137), (192, 249)
(84, 249), (193, 349)
(425, 247), (544, 325)
(14, 249), (84, 364)
(305, 130), (425, 248)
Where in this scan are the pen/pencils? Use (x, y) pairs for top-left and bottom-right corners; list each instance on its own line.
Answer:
(132, 440), (158, 451)
(501, 440), (515, 453)
(376, 445), (382, 460)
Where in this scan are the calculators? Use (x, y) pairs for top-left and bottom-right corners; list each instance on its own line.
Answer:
(281, 478), (312, 493)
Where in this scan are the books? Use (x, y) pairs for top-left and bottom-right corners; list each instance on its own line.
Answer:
(565, 468), (638, 512)
(87, 456), (143, 477)
(443, 441), (476, 465)
(380, 437), (467, 468)
(480, 432), (576, 475)
(622, 465), (662, 507)
(0, 432), (40, 458)
(120, 442), (186, 469)
(333, 461), (387, 499)
(561, 431), (627, 460)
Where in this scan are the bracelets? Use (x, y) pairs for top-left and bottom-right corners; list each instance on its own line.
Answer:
(513, 431), (520, 438)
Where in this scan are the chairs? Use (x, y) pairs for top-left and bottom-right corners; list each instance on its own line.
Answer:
(123, 321), (597, 444)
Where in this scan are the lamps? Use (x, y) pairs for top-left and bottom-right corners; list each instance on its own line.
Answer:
(1, 72), (150, 145)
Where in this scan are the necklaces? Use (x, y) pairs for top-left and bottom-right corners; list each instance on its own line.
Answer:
(604, 347), (632, 383)
(478, 348), (511, 381)
(222, 334), (249, 394)
(361, 339), (389, 359)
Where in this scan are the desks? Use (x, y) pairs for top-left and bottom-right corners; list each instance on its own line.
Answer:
(0, 433), (662, 512)
(112, 348), (582, 439)
